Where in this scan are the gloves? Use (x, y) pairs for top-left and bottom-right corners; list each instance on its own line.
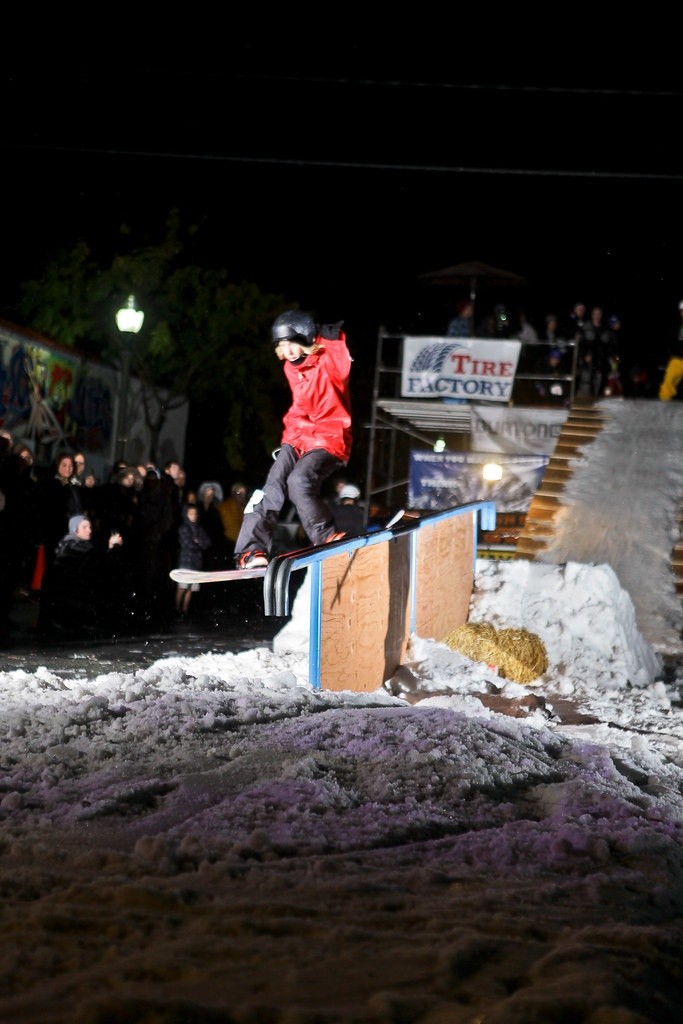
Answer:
(318, 320), (345, 340)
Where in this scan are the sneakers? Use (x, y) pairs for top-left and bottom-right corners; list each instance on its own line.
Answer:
(233, 549), (269, 569)
(323, 530), (349, 544)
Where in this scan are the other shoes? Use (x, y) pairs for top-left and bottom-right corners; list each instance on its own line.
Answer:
(174, 609), (191, 623)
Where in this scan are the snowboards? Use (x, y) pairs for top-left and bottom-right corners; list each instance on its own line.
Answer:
(166, 566), (268, 585)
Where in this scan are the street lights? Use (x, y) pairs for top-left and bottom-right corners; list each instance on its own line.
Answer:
(106, 292), (146, 486)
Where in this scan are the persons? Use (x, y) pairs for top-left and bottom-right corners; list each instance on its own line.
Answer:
(658, 301), (683, 402)
(445, 295), (624, 404)
(233, 308), (354, 570)
(0, 429), (381, 652)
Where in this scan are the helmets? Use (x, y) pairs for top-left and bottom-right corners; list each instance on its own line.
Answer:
(339, 484), (361, 499)
(271, 309), (317, 346)
